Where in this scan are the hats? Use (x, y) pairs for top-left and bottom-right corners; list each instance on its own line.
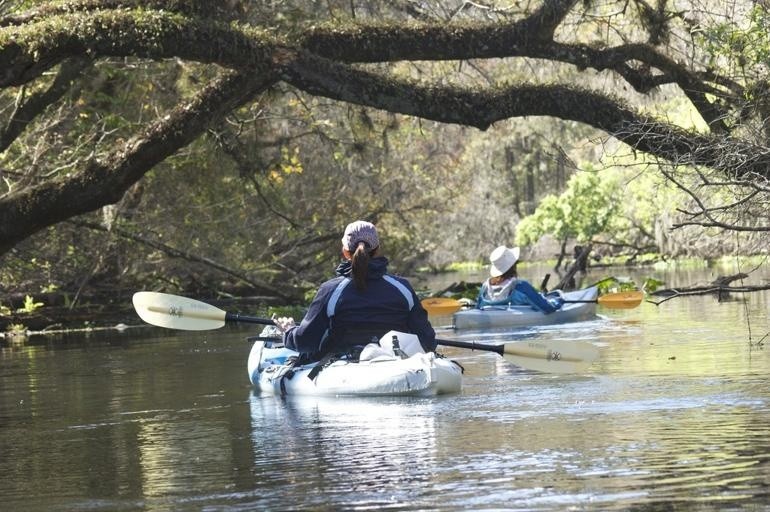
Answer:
(340, 220), (380, 250)
(489, 246), (521, 278)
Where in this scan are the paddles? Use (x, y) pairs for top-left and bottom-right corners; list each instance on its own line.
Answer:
(132, 291), (599, 376)
(421, 287), (644, 317)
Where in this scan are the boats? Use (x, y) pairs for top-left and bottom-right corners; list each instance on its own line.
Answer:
(247, 312), (462, 399)
(450, 281), (602, 329)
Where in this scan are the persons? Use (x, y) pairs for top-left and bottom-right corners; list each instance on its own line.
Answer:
(274, 220), (439, 366)
(477, 246), (565, 314)
